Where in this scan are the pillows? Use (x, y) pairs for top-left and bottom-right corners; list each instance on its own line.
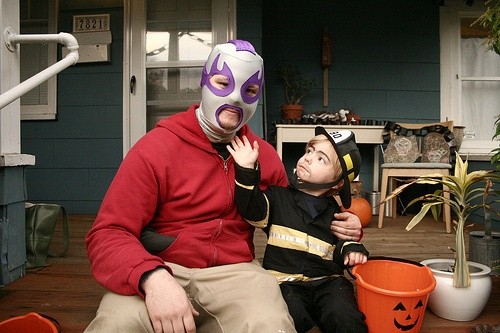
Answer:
(381, 120), (456, 164)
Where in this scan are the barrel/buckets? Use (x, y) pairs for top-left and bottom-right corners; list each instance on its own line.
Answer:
(0, 311), (61, 333)
(347, 255), (436, 333)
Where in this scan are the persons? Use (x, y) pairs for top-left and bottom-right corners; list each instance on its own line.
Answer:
(226, 126), (370, 333)
(84, 40), (363, 333)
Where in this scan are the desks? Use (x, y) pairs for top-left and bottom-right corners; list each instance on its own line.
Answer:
(275, 123), (385, 192)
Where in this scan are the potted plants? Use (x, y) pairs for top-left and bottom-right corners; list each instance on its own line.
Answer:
(278, 63), (317, 125)
(374, 149), (492, 321)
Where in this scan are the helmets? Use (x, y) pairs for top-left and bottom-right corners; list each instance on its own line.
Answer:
(316, 126), (361, 209)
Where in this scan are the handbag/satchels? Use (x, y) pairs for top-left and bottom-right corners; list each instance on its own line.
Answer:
(24, 203), (69, 269)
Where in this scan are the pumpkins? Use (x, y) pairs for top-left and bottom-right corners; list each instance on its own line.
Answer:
(341, 189), (372, 228)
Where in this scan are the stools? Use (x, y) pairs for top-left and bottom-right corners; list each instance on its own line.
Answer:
(469, 230), (500, 276)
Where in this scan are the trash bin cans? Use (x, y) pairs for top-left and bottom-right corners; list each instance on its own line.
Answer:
(366, 190), (381, 216)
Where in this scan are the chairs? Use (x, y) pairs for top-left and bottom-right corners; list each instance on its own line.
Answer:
(377, 121), (453, 234)
